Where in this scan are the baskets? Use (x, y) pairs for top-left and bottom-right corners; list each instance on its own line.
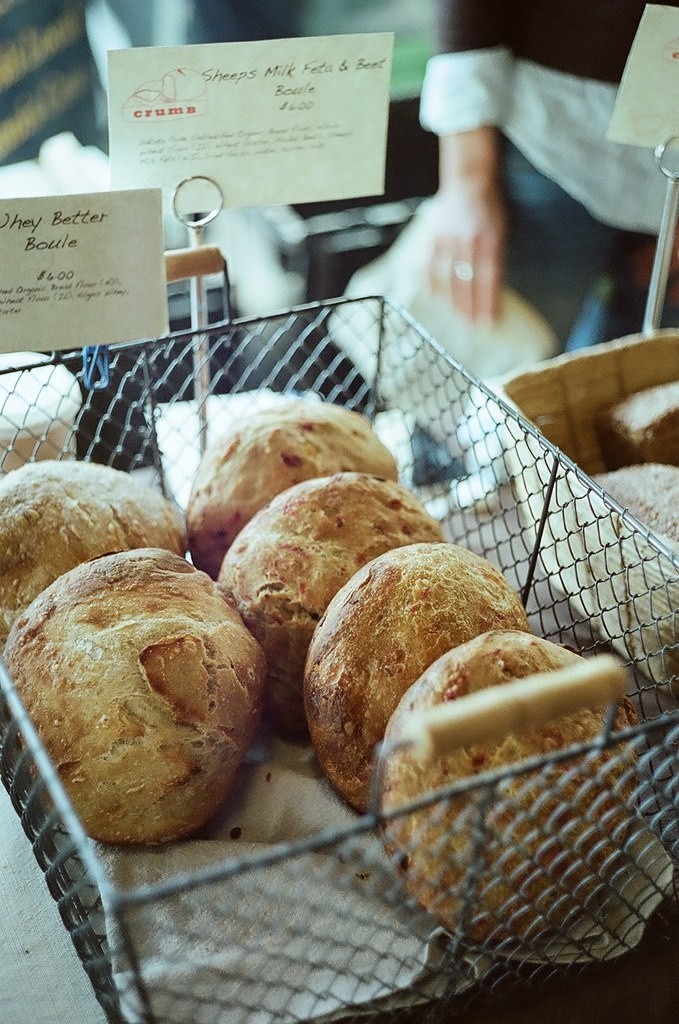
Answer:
(0, 293), (679, 1024)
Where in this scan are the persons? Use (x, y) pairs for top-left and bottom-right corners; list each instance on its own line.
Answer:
(417, 0), (679, 354)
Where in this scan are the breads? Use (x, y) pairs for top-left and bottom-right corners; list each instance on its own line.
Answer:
(0, 401), (637, 941)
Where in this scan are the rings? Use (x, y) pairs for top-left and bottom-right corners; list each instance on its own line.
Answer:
(450, 262), (475, 282)
